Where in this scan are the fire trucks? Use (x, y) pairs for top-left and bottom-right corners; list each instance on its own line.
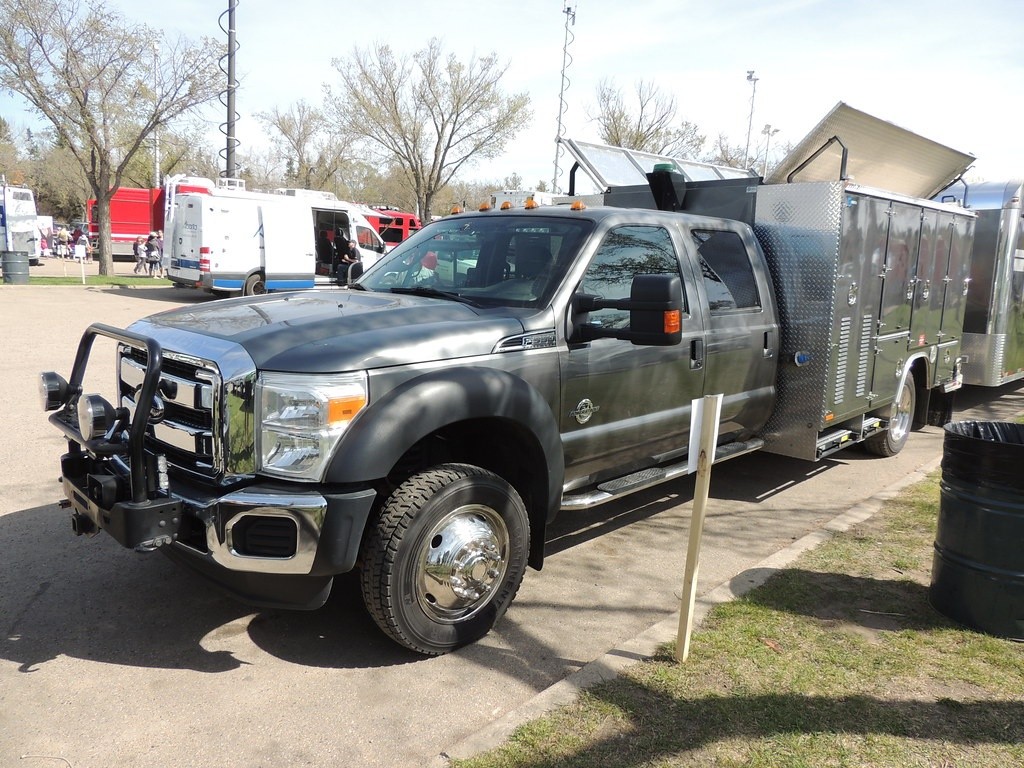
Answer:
(84, 172), (430, 263)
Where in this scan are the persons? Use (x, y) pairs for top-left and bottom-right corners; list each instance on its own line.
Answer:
(134, 230), (164, 279)
(338, 240), (363, 286)
(56, 225), (91, 264)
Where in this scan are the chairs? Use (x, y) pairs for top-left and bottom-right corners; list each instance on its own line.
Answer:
(513, 244), (553, 278)
(332, 228), (349, 251)
(317, 230), (336, 263)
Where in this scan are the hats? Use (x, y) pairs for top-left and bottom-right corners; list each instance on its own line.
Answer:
(347, 240), (356, 244)
(149, 232), (159, 236)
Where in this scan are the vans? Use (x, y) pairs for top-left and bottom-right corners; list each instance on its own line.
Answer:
(159, 172), (390, 302)
(0, 174), (43, 266)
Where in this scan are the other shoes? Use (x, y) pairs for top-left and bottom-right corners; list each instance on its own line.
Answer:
(153, 276), (160, 279)
(134, 268), (138, 274)
(161, 274), (164, 278)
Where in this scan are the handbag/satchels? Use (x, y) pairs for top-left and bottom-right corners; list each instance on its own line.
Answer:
(148, 251), (161, 261)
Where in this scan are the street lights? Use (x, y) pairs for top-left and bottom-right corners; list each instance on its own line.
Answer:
(744, 69), (759, 169)
(761, 124), (779, 182)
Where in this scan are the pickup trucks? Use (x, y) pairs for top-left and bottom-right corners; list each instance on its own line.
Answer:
(34, 101), (986, 660)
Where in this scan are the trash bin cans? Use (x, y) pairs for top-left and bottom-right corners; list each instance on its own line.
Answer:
(928, 421), (1024, 642)
(1, 250), (30, 284)
